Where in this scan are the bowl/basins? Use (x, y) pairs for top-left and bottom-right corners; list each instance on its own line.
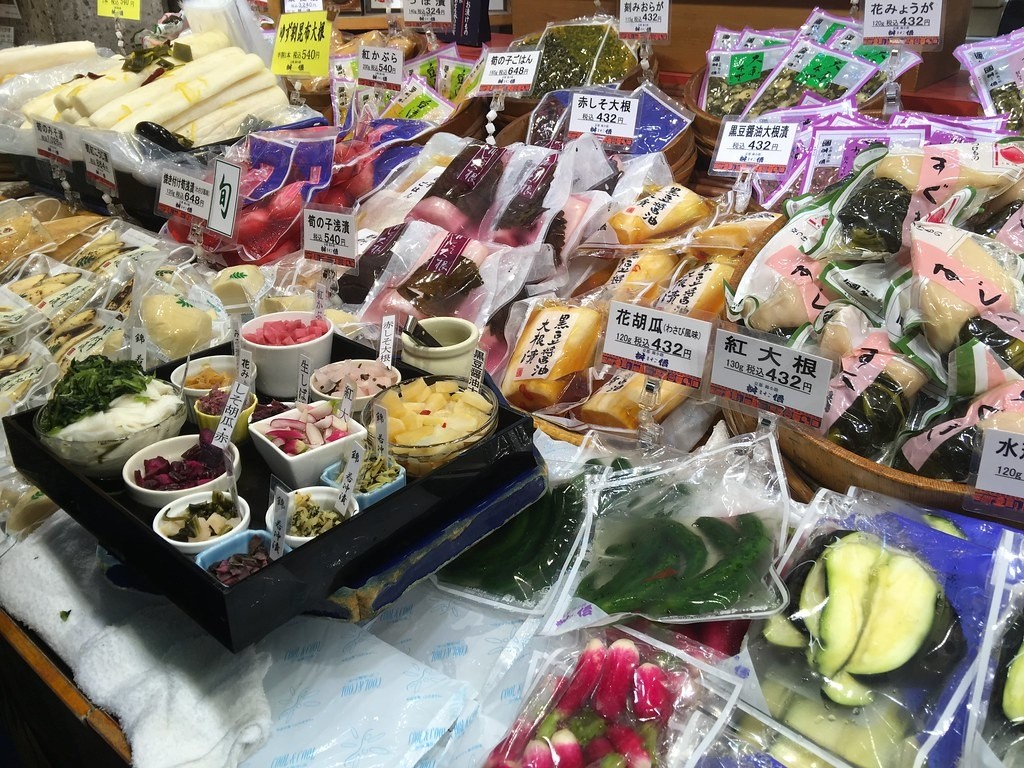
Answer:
(309, 359), (402, 424)
(319, 453), (407, 512)
(248, 400), (367, 489)
(151, 490), (251, 562)
(265, 485), (359, 549)
(32, 377), (188, 497)
(193, 386), (259, 452)
(121, 434), (243, 528)
(195, 530), (293, 588)
(170, 355), (258, 424)
(401, 316), (480, 378)
(360, 374), (499, 486)
(238, 311), (334, 398)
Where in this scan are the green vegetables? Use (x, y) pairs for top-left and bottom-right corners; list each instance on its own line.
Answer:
(35, 353), (158, 437)
(161, 490), (238, 542)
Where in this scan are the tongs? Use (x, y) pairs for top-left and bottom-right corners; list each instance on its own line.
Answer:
(402, 314), (442, 347)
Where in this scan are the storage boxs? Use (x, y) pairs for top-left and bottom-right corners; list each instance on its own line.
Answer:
(3, 332), (535, 653)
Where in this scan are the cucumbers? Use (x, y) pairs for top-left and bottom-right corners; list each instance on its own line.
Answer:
(437, 454), (639, 597)
(576, 512), (774, 614)
